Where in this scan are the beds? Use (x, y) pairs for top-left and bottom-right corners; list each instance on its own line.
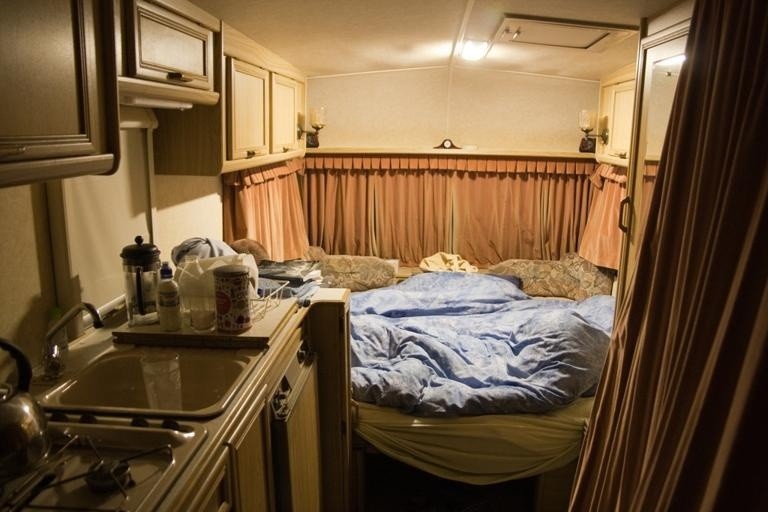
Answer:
(292, 246), (618, 485)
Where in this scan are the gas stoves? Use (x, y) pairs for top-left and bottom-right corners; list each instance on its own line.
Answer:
(1, 411), (211, 512)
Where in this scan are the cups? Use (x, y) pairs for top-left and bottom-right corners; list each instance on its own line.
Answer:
(212, 265), (255, 333)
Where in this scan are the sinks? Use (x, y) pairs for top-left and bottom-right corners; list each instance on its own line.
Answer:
(35, 341), (266, 419)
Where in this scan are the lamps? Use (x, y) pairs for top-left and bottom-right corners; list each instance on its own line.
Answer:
(296, 105), (327, 146)
(575, 107), (609, 155)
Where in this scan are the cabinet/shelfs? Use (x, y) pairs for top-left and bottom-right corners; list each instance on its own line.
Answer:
(1, 0), (125, 191)
(152, 18), (305, 178)
(593, 64), (639, 168)
(116, 0), (220, 111)
(275, 334), (332, 509)
(202, 389), (276, 511)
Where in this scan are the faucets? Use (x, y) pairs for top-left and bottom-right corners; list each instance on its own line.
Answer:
(44, 301), (102, 352)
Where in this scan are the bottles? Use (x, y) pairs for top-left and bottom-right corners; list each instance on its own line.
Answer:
(181, 255), (216, 331)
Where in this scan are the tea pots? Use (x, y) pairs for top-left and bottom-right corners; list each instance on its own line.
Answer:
(0, 338), (51, 477)
(121, 235), (164, 326)
